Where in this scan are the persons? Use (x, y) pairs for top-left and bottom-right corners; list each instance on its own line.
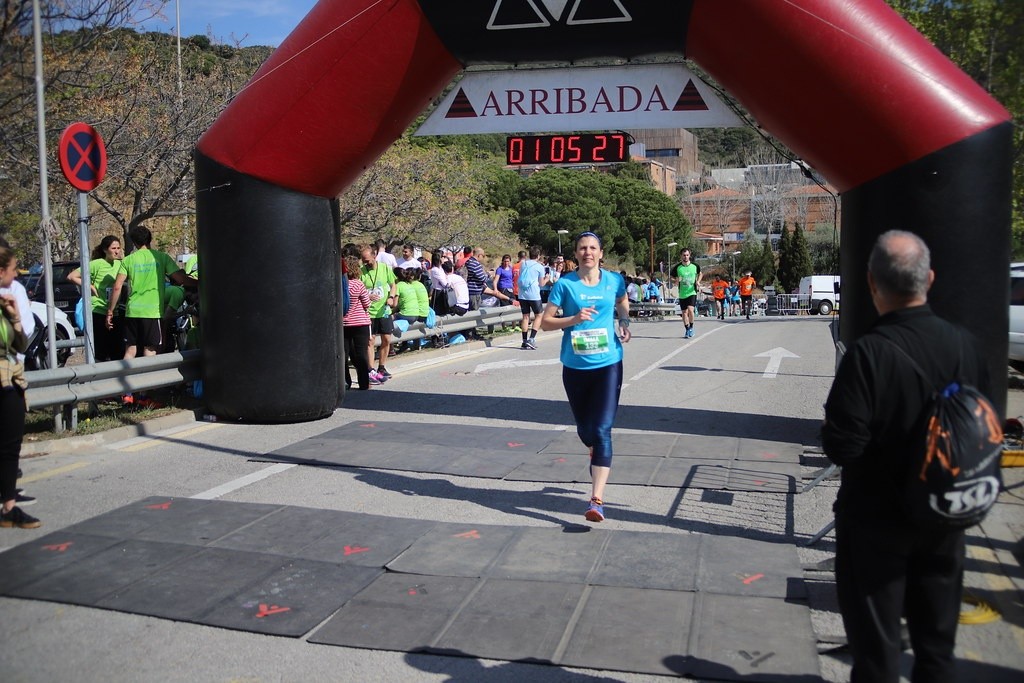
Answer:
(821, 229), (994, 683)
(67, 225), (198, 408)
(341, 239), (566, 391)
(620, 271), (660, 317)
(712, 270), (756, 320)
(0, 236), (41, 528)
(671, 248), (703, 338)
(542, 231), (632, 522)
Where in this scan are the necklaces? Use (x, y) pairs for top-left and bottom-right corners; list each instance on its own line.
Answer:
(366, 266), (378, 288)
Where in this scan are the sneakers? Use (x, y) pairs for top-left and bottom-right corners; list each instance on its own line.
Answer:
(368, 374), (382, 385)
(685, 327), (694, 339)
(122, 394), (134, 406)
(522, 343), (531, 350)
(525, 339), (537, 350)
(377, 367), (391, 378)
(371, 369), (389, 381)
(138, 398), (162, 410)
(585, 501), (604, 522)
(102, 396), (117, 405)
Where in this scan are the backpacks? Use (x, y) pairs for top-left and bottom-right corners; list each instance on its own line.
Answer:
(456, 260), (478, 282)
(518, 261), (538, 290)
(873, 331), (1006, 525)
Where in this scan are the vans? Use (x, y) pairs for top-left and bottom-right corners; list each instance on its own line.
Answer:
(799, 275), (840, 314)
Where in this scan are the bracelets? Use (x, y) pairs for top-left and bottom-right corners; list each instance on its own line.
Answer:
(619, 318), (629, 327)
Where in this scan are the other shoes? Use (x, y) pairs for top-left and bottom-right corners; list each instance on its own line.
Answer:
(704, 310), (750, 320)
(503, 326), (512, 332)
(0, 506), (41, 528)
(15, 488), (24, 495)
(376, 332), (483, 359)
(514, 326), (522, 332)
(15, 494), (38, 504)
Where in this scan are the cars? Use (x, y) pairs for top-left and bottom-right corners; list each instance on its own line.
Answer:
(29, 301), (77, 370)
(1009, 262), (1024, 361)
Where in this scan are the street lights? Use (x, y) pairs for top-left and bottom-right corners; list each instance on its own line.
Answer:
(668, 242), (677, 298)
(732, 251), (741, 284)
(558, 230), (568, 255)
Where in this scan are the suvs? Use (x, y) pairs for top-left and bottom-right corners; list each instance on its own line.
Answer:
(766, 296), (787, 316)
(38, 260), (81, 324)
